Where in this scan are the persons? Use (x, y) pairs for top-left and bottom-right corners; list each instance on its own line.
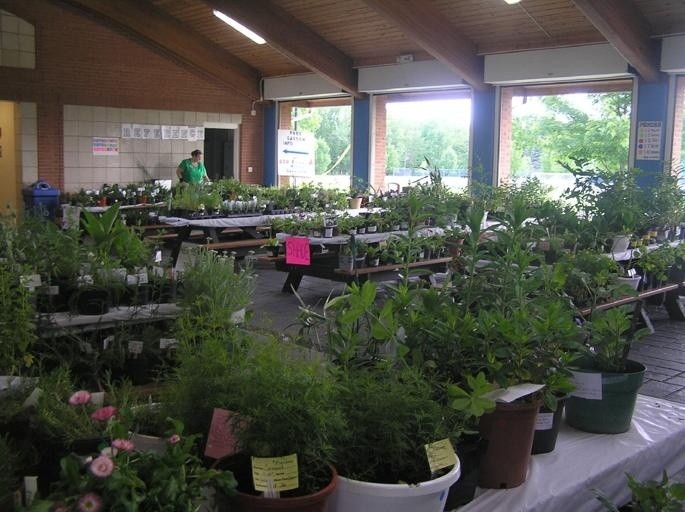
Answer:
(177, 150), (210, 184)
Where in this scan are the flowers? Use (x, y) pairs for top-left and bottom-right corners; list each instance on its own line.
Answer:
(64, 438), (159, 512)
(26, 364), (133, 453)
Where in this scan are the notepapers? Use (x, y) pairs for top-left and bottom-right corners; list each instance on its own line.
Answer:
(424, 438), (457, 474)
(251, 453), (298, 491)
(204, 408), (254, 463)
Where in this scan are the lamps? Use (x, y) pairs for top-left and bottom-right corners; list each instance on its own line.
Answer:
(212, 8), (268, 45)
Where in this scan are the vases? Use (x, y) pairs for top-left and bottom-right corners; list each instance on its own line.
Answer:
(68, 436), (106, 472)
(135, 467), (155, 494)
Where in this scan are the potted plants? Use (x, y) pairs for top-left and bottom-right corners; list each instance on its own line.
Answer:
(165, 148), (684, 331)
(286, 281), (461, 511)
(387, 268), (570, 490)
(397, 191), (570, 455)
(325, 281), (487, 512)
(0, 208), (251, 394)
(188, 335), (337, 512)
(0, 267), (39, 400)
(20, 412), (58, 508)
(65, 181), (167, 230)
(563, 269), (646, 433)
(108, 258), (259, 469)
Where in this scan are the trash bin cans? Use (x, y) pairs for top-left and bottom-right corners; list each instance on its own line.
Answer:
(22, 181), (61, 226)
